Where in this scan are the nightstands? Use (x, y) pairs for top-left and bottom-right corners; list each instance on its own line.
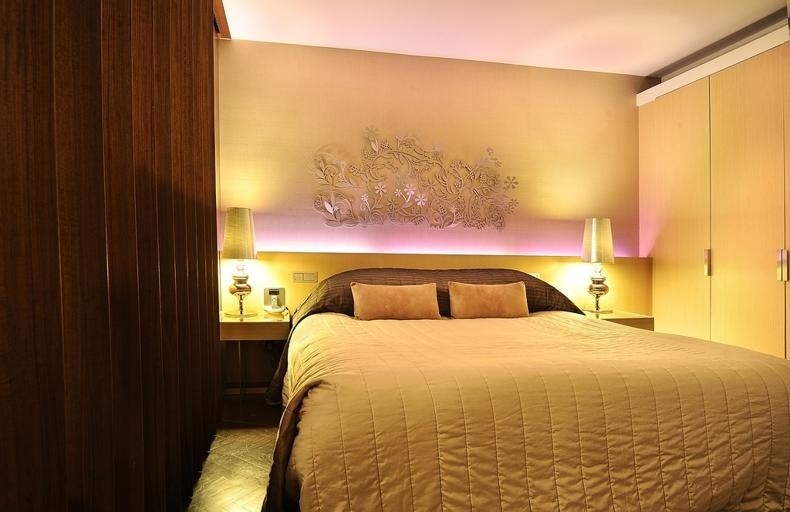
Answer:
(220, 310), (291, 412)
(601, 310), (654, 332)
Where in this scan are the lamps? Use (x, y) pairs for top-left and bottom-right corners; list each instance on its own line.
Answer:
(583, 218), (614, 312)
(222, 207), (258, 317)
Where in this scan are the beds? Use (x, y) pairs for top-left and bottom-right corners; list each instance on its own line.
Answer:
(263, 312), (790, 512)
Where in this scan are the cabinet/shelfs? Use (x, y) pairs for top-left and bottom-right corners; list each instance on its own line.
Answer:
(636, 76), (709, 340)
(709, 41), (790, 361)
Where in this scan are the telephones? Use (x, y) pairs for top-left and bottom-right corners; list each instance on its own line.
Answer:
(264, 295), (286, 314)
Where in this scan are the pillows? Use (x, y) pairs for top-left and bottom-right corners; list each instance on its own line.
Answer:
(350, 281), (443, 321)
(448, 281), (529, 319)
(292, 268), (585, 331)
